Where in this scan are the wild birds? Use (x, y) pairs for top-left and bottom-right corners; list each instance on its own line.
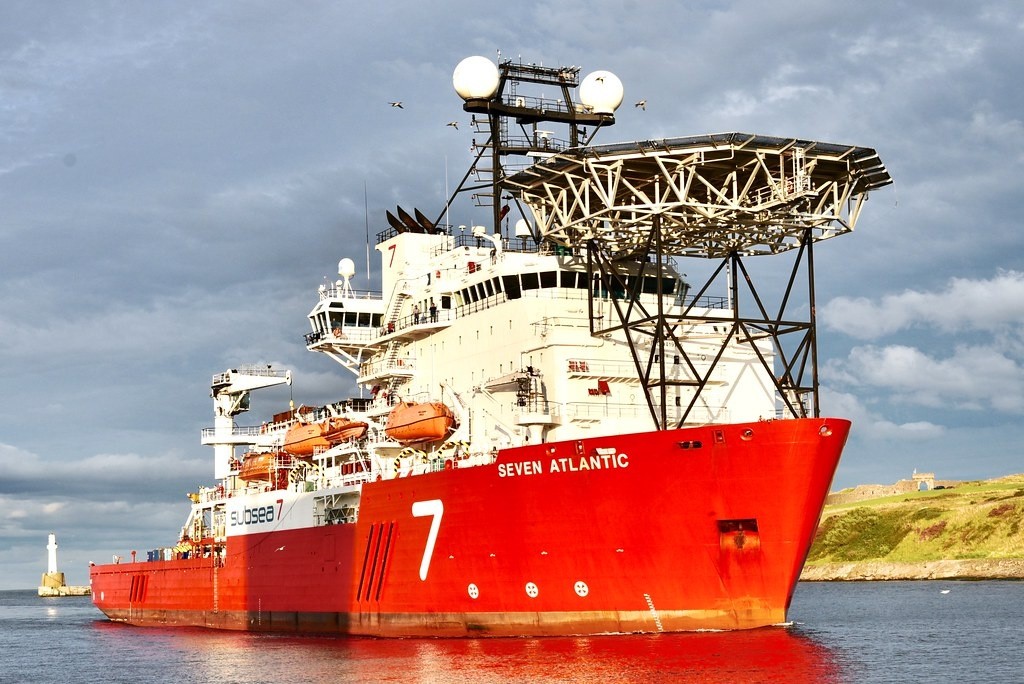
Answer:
(595, 76), (604, 83)
(446, 122), (458, 130)
(388, 102), (404, 109)
(635, 100), (647, 111)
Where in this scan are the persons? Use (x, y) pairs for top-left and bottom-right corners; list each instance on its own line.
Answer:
(413, 305), (420, 324)
(214, 483), (224, 498)
(379, 321), (393, 337)
(240, 455), (244, 462)
(429, 303), (437, 323)
(230, 457), (234, 470)
(331, 318), (339, 333)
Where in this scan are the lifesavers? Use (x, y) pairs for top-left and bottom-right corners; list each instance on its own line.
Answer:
(334, 327), (342, 338)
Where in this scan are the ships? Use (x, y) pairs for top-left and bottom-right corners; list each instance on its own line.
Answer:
(87, 50), (892, 634)
